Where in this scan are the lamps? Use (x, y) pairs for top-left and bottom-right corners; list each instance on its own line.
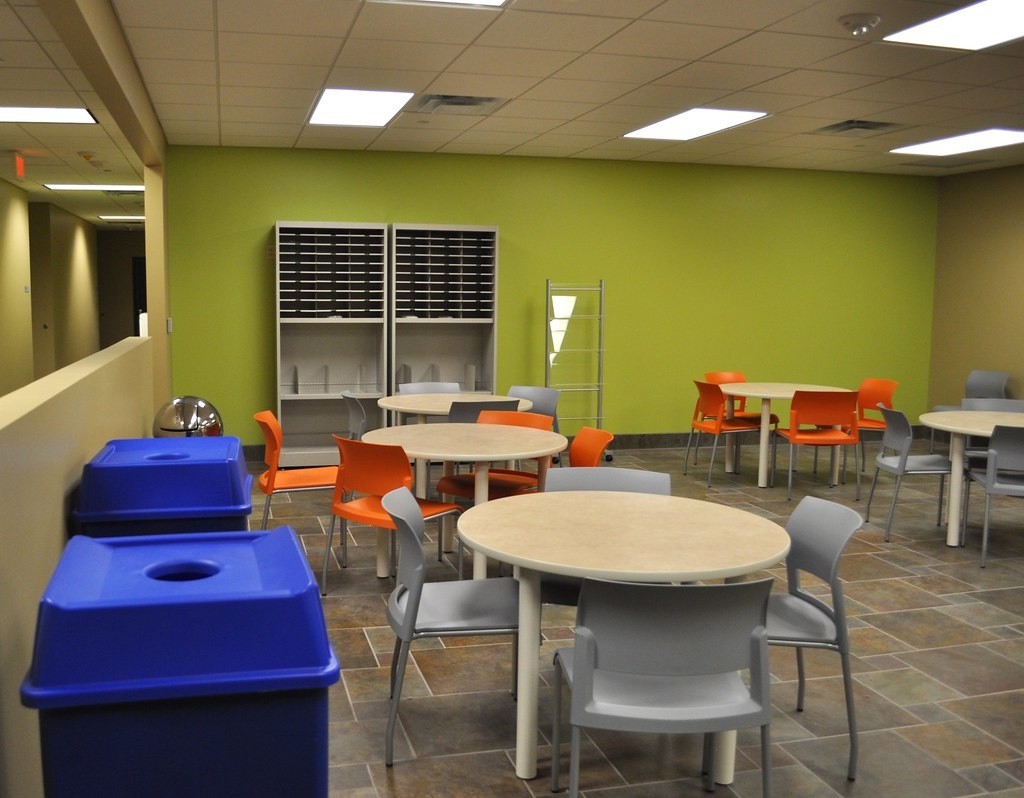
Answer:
(138, 309), (148, 338)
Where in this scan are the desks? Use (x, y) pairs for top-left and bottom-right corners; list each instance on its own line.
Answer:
(918, 411), (1024, 546)
(716, 382), (853, 488)
(454, 490), (791, 798)
(376, 392), (536, 501)
(359, 423), (569, 582)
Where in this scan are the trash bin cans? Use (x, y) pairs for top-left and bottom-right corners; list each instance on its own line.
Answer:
(18, 521), (342, 798)
(80, 435), (251, 536)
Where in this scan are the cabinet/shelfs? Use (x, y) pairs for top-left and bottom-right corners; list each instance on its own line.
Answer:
(391, 223), (498, 428)
(275, 221), (388, 466)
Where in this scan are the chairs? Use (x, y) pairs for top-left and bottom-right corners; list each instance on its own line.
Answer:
(684, 370), (1024, 569)
(252, 409), (351, 569)
(378, 485), (522, 767)
(549, 575), (775, 798)
(320, 433), (465, 599)
(339, 380), (672, 610)
(767, 496), (864, 782)
